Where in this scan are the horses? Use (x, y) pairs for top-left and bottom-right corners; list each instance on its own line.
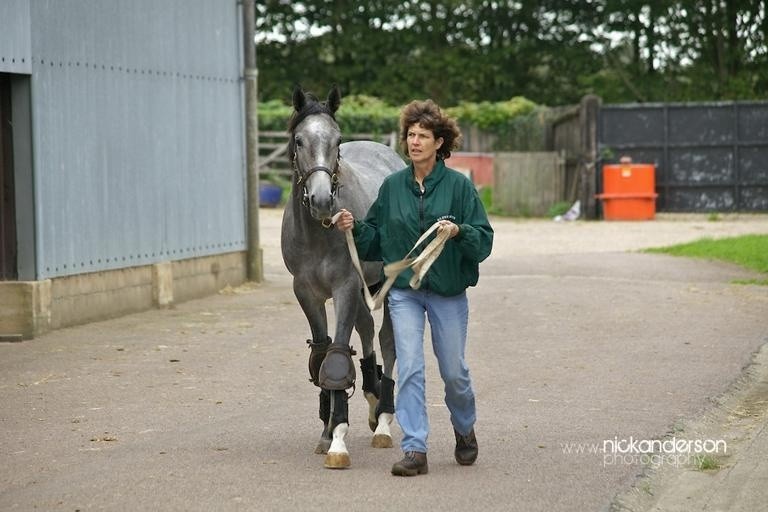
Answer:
(280, 82), (409, 470)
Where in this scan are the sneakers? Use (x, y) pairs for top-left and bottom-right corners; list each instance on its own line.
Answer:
(454, 422), (479, 466)
(390, 450), (430, 477)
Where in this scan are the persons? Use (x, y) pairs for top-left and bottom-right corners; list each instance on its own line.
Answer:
(336, 97), (493, 479)
